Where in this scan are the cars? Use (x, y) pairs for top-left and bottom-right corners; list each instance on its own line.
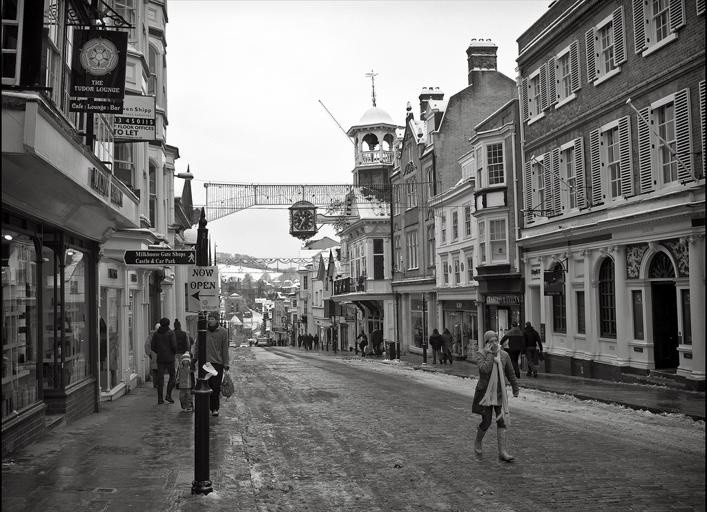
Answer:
(229, 339), (269, 347)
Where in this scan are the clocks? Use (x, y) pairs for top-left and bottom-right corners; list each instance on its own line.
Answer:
(288, 201), (318, 240)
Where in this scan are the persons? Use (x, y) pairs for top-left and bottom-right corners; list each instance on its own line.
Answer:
(143, 317), (194, 404)
(441, 328), (453, 364)
(429, 328), (442, 364)
(176, 351), (196, 413)
(499, 320), (523, 378)
(296, 333), (319, 351)
(370, 327), (383, 357)
(356, 329), (368, 357)
(470, 330), (519, 461)
(521, 322), (542, 378)
(191, 311), (230, 416)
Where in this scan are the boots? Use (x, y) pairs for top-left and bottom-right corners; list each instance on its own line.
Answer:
(474, 424), (487, 455)
(526, 369), (537, 378)
(497, 428), (515, 461)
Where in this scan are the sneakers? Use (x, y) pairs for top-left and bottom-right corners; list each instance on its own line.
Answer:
(212, 409), (219, 416)
(181, 402), (193, 412)
(158, 396), (174, 404)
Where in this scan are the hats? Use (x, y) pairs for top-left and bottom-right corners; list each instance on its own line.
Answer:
(181, 351), (191, 365)
(484, 330), (499, 345)
(155, 317), (181, 327)
(208, 311), (220, 320)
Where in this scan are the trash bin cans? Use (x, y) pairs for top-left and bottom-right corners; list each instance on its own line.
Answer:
(383, 340), (399, 359)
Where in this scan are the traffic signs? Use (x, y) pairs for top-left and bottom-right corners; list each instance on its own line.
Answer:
(122, 249), (195, 264)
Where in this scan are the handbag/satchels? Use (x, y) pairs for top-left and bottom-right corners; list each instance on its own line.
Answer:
(533, 348), (545, 365)
(502, 334), (509, 348)
(222, 375), (234, 397)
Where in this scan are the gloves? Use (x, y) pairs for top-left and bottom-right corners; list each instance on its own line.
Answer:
(513, 392), (520, 398)
